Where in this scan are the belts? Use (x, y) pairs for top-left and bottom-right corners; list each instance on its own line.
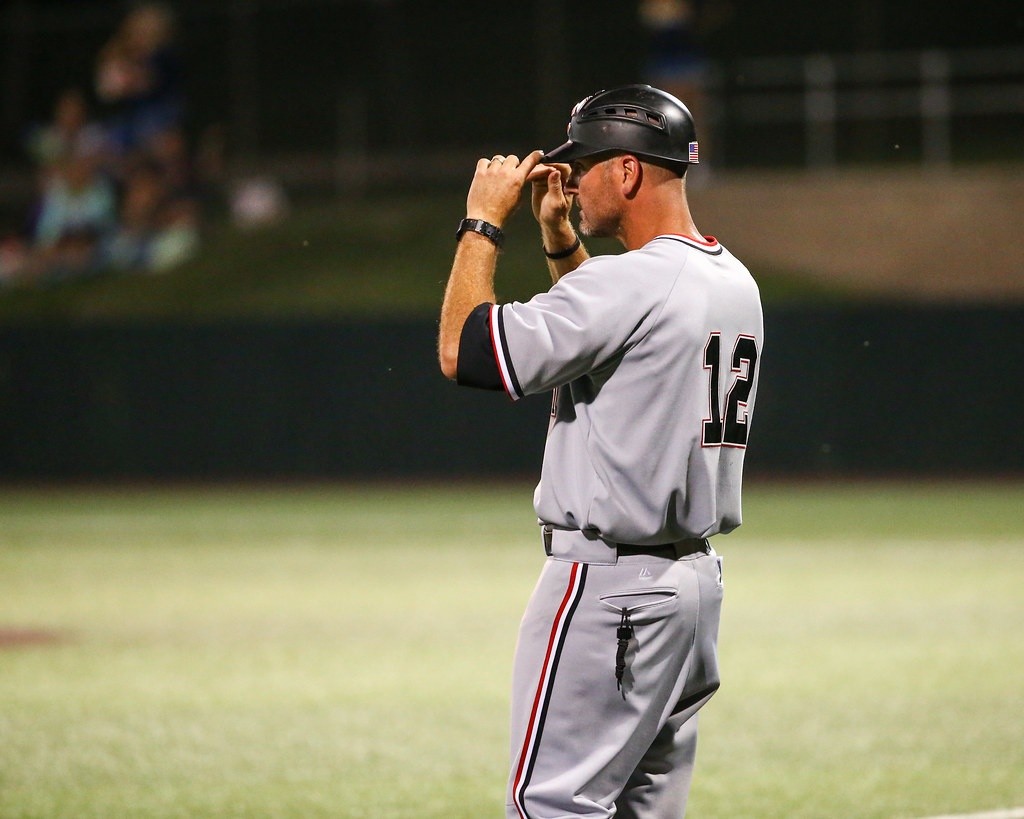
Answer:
(542, 526), (707, 560)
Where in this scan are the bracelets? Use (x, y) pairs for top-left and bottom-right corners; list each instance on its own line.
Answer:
(456, 218), (506, 250)
(543, 233), (581, 258)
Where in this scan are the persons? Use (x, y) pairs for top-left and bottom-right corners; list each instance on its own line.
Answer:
(437, 82), (763, 818)
(31, 8), (290, 281)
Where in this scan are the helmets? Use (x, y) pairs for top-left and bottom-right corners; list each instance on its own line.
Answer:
(538, 85), (698, 166)
